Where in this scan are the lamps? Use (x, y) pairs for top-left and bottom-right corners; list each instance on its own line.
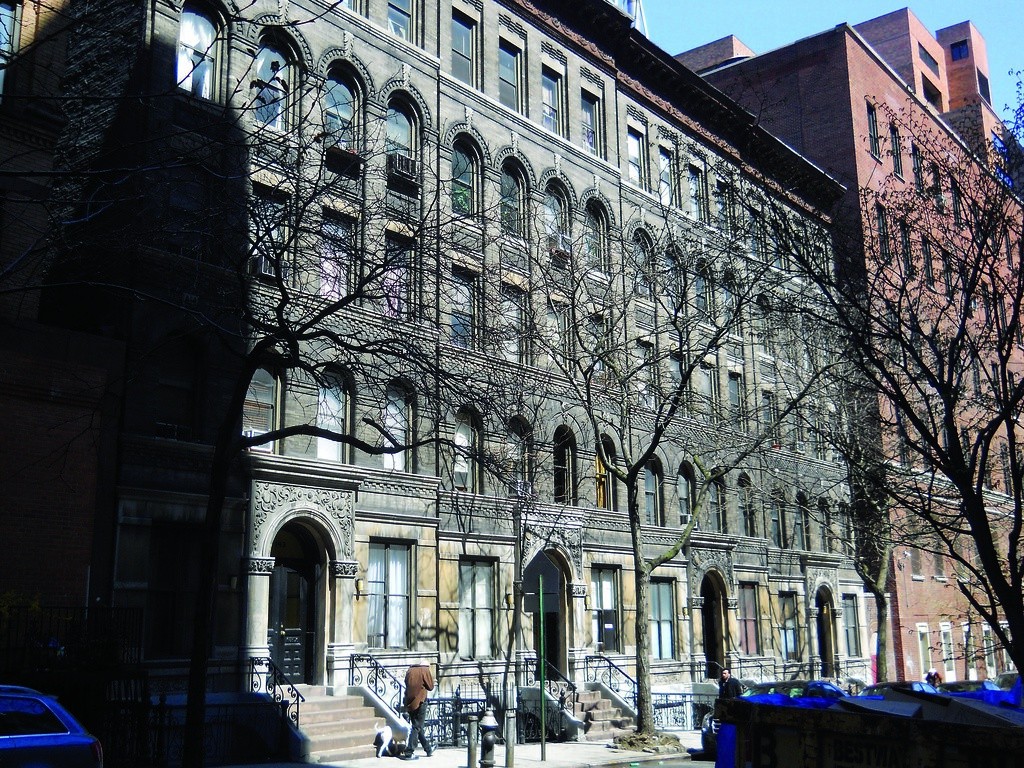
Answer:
(506, 593), (513, 605)
(682, 607), (689, 617)
(585, 595), (591, 606)
(356, 578), (364, 592)
(735, 609), (741, 616)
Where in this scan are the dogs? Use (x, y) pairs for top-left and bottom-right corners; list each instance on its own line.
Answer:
(374, 721), (414, 758)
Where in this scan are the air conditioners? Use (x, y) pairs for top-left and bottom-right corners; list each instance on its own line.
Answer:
(790, 441), (806, 455)
(506, 479), (532, 498)
(386, 152), (417, 182)
(242, 428), (274, 454)
(633, 282), (650, 298)
(549, 233), (574, 257)
(251, 254), (290, 283)
(679, 513), (698, 529)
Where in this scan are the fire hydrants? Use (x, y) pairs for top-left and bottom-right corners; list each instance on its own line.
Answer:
(478, 706), (499, 768)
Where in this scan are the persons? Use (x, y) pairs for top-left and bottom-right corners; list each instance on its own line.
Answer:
(399, 660), (438, 761)
(925, 670), (943, 689)
(718, 668), (743, 700)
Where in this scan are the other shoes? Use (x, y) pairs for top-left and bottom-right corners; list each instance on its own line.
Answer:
(403, 754), (419, 760)
(427, 744), (437, 757)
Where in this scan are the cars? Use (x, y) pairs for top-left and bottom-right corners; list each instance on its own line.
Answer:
(934, 680), (1001, 693)
(992, 671), (1021, 691)
(701, 679), (851, 761)
(857, 680), (938, 700)
(0, 684), (103, 768)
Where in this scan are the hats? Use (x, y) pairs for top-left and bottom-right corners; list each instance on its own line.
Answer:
(420, 660), (430, 666)
(928, 669), (936, 672)
